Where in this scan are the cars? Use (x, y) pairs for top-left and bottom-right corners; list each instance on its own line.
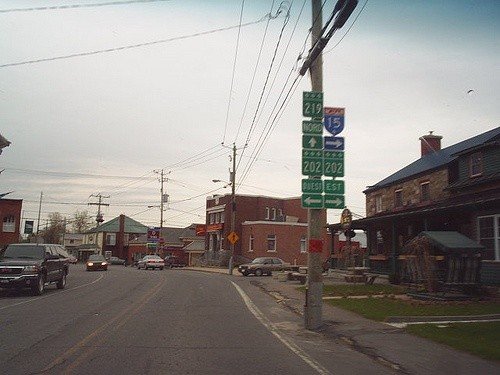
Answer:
(108, 257), (126, 265)
(162, 256), (186, 268)
(137, 254), (166, 270)
(85, 254), (108, 271)
(238, 256), (290, 277)
(67, 254), (78, 264)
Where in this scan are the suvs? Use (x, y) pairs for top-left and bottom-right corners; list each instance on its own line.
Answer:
(0, 242), (71, 296)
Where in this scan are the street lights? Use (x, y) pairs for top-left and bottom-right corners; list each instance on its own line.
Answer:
(148, 205), (163, 252)
(212, 179), (236, 275)
